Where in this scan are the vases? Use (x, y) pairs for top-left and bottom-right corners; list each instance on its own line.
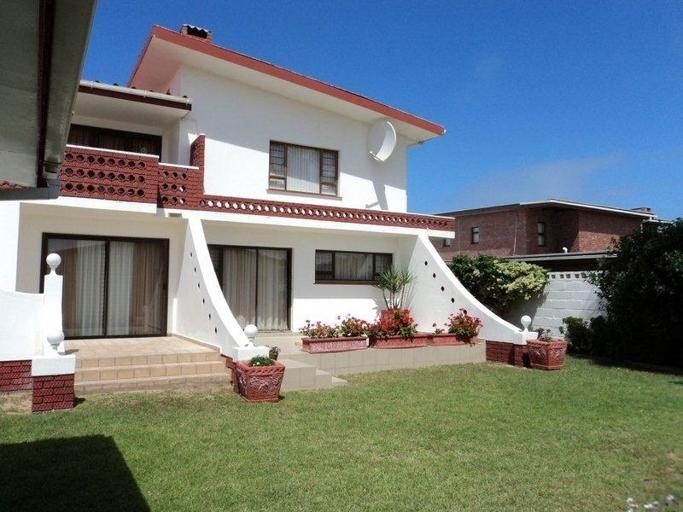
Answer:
(302, 337), (370, 352)
(527, 338), (567, 371)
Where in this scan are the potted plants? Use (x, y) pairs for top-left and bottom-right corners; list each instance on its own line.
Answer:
(235, 354), (285, 403)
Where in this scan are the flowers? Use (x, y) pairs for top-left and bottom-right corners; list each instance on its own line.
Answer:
(300, 308), (483, 346)
